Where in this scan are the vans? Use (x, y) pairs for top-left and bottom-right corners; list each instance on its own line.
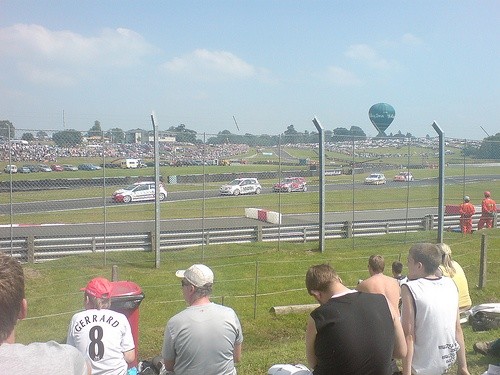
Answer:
(121, 159), (142, 169)
(4, 164), (17, 173)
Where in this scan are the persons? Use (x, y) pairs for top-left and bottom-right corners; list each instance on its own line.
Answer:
(268, 191), (498, 375)
(0, 253), (92, 375)
(154, 264), (243, 375)
(68, 277), (136, 375)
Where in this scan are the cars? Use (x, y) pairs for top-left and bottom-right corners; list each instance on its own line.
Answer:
(394, 172), (414, 182)
(21, 167), (30, 172)
(51, 164), (63, 171)
(273, 176), (308, 193)
(62, 164), (78, 171)
(78, 163), (102, 171)
(364, 173), (387, 185)
(219, 177), (261, 196)
(112, 181), (167, 203)
(38, 164), (51, 172)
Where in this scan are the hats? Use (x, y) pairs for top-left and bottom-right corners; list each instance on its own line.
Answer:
(464, 196), (470, 201)
(80, 278), (112, 300)
(484, 191), (491, 195)
(176, 264), (214, 288)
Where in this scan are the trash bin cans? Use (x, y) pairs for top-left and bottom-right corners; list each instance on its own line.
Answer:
(109, 281), (144, 371)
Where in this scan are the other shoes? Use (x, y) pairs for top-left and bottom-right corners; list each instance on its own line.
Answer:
(474, 337), (500, 355)
